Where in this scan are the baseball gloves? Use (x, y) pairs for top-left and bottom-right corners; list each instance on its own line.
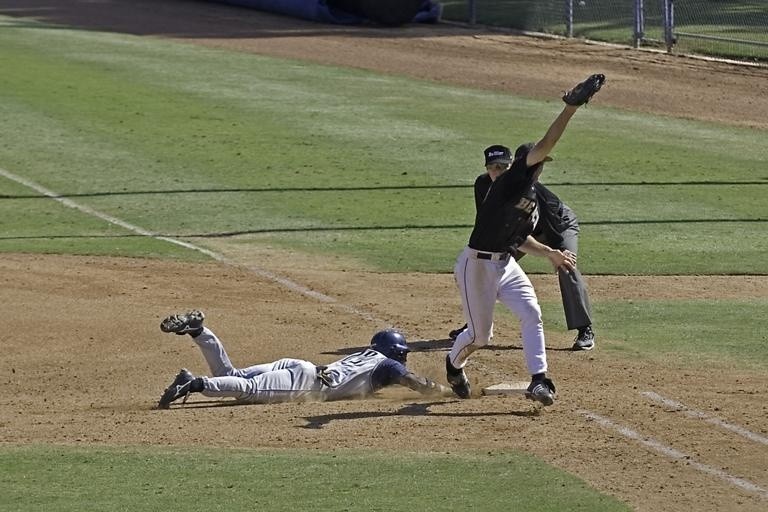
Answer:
(562, 74), (605, 106)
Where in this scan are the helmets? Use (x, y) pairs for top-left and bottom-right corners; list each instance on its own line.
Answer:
(370, 330), (411, 362)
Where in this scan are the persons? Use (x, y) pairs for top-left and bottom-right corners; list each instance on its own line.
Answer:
(153, 309), (454, 407)
(447, 144), (598, 352)
(446, 74), (607, 406)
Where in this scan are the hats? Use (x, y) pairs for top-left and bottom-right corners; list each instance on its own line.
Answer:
(514, 142), (553, 162)
(483, 145), (513, 166)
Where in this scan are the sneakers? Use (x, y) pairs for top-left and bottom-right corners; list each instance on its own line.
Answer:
(571, 325), (595, 352)
(159, 308), (206, 335)
(445, 354), (473, 399)
(526, 377), (556, 406)
(156, 367), (196, 409)
(449, 323), (468, 340)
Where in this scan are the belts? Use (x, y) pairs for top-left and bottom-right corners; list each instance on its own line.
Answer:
(477, 252), (508, 261)
